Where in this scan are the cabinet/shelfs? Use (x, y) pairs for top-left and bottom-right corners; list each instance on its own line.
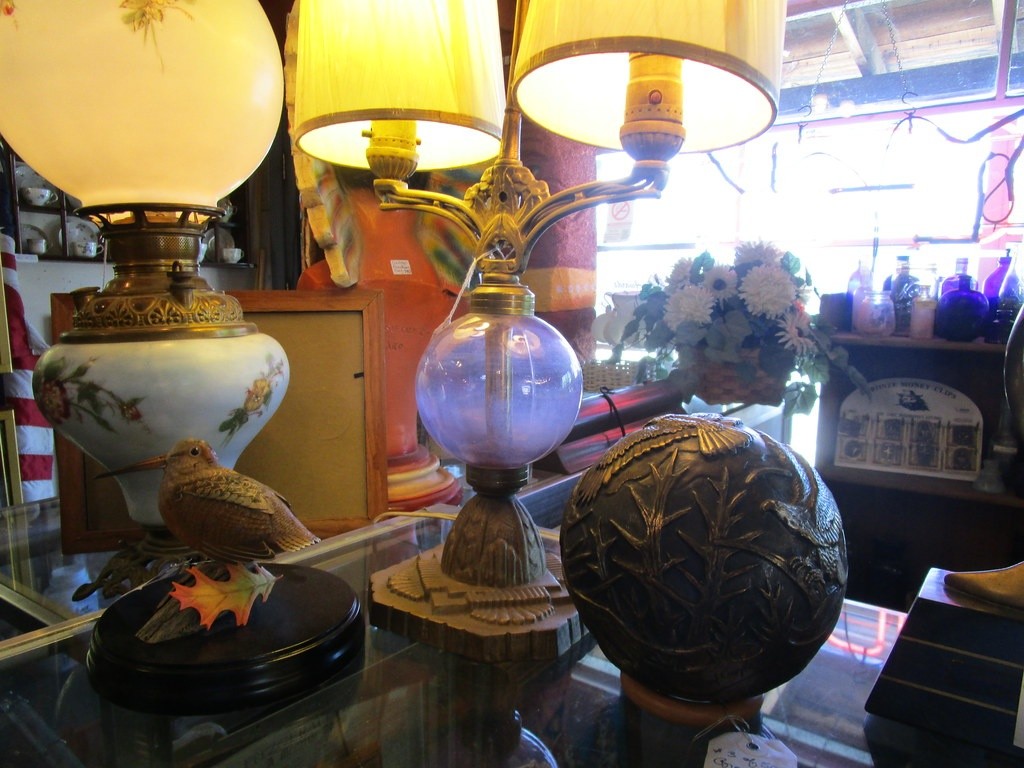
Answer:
(11, 146), (254, 268)
(816, 293), (1024, 503)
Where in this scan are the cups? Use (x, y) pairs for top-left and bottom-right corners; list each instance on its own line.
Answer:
(221, 248), (244, 263)
(27, 238), (47, 255)
(19, 188), (58, 206)
(71, 240), (103, 259)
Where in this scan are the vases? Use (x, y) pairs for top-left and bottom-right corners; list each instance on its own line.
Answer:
(676, 342), (794, 407)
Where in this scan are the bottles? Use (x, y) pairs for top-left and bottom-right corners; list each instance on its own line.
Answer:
(853, 254), (1022, 345)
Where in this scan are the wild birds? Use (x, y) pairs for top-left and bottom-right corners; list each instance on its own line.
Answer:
(94, 438), (321, 580)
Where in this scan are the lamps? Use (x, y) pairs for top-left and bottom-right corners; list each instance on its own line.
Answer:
(284, 0), (788, 670)
(0, 0), (290, 601)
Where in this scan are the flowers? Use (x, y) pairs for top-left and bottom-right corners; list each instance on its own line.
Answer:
(603, 242), (874, 416)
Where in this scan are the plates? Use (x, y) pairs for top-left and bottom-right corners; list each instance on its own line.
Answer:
(16, 165), (57, 192)
(203, 227), (235, 262)
(57, 219), (101, 256)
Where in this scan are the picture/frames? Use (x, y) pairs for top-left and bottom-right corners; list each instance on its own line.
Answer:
(49, 289), (391, 556)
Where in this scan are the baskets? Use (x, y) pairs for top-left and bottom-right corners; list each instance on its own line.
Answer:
(583, 361), (643, 394)
(676, 345), (796, 406)
